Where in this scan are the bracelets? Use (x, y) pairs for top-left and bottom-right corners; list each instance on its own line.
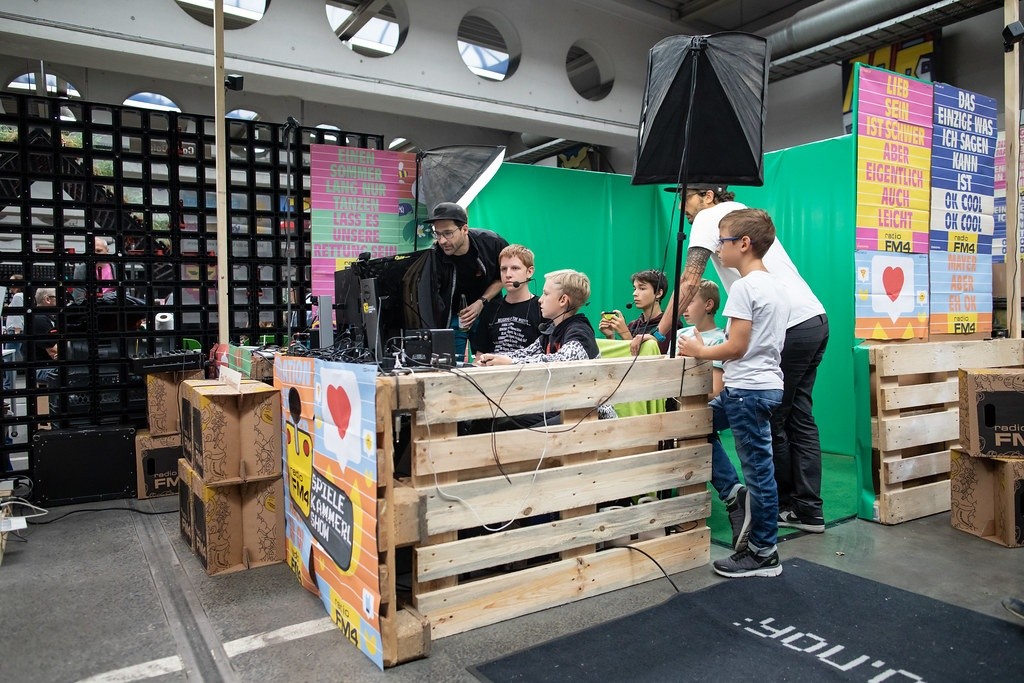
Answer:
(478, 296), (489, 306)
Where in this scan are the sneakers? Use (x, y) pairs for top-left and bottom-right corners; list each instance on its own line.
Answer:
(726, 487), (755, 552)
(713, 540), (784, 578)
(777, 505), (825, 533)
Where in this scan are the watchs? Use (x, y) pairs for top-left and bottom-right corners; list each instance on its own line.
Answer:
(650, 327), (666, 343)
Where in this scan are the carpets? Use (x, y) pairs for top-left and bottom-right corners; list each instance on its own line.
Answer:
(463, 557), (1024, 682)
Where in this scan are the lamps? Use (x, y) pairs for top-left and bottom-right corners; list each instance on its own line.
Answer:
(1002, 21), (1024, 53)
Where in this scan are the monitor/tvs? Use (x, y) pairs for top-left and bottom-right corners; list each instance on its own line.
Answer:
(318, 248), (440, 364)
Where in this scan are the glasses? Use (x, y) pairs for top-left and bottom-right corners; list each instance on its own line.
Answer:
(433, 226), (463, 239)
(717, 237), (742, 245)
(678, 191), (706, 202)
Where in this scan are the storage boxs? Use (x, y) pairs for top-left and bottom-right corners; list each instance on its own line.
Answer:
(950, 368), (1024, 548)
(134, 367), (286, 576)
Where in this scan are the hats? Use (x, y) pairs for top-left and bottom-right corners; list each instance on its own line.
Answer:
(664, 183), (728, 193)
(422, 202), (468, 224)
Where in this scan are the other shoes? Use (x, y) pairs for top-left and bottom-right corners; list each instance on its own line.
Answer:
(1001, 597), (1024, 619)
(50, 422), (60, 430)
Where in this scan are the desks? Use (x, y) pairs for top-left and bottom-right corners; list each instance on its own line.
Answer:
(2, 349), (18, 437)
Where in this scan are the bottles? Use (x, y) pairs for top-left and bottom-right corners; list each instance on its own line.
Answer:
(457, 294), (472, 332)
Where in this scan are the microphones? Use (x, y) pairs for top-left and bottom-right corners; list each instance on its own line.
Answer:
(513, 278), (533, 289)
(626, 301), (635, 309)
(538, 307), (574, 333)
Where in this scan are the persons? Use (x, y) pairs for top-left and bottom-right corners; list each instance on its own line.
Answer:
(476, 246), (554, 354)
(34, 287), (59, 384)
(73, 237), (129, 305)
(479, 269), (619, 444)
(598, 268), (673, 536)
(3, 275), (24, 390)
(664, 180), (828, 578)
(423, 201), (528, 436)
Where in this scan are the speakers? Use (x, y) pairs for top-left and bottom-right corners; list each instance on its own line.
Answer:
(225, 74), (243, 90)
